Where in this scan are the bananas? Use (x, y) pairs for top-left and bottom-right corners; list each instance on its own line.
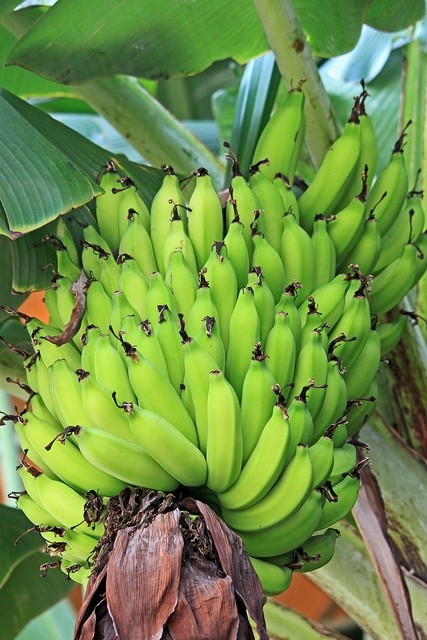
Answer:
(10, 83), (427, 596)
